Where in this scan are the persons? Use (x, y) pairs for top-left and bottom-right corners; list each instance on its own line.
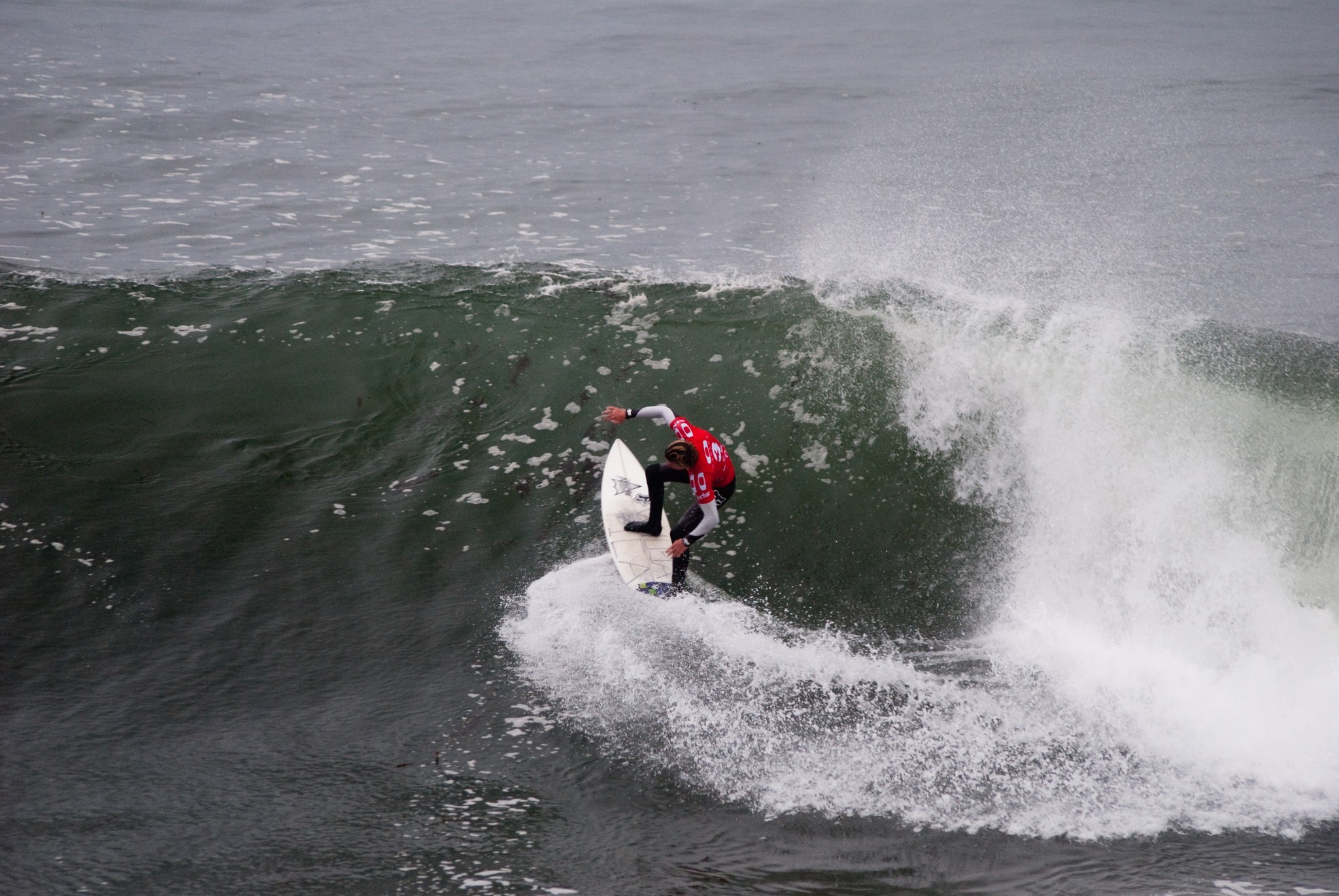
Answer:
(602, 402), (737, 598)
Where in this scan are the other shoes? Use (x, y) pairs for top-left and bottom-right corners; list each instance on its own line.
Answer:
(624, 520), (662, 537)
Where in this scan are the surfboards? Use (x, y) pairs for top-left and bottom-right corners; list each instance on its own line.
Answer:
(600, 439), (677, 599)
